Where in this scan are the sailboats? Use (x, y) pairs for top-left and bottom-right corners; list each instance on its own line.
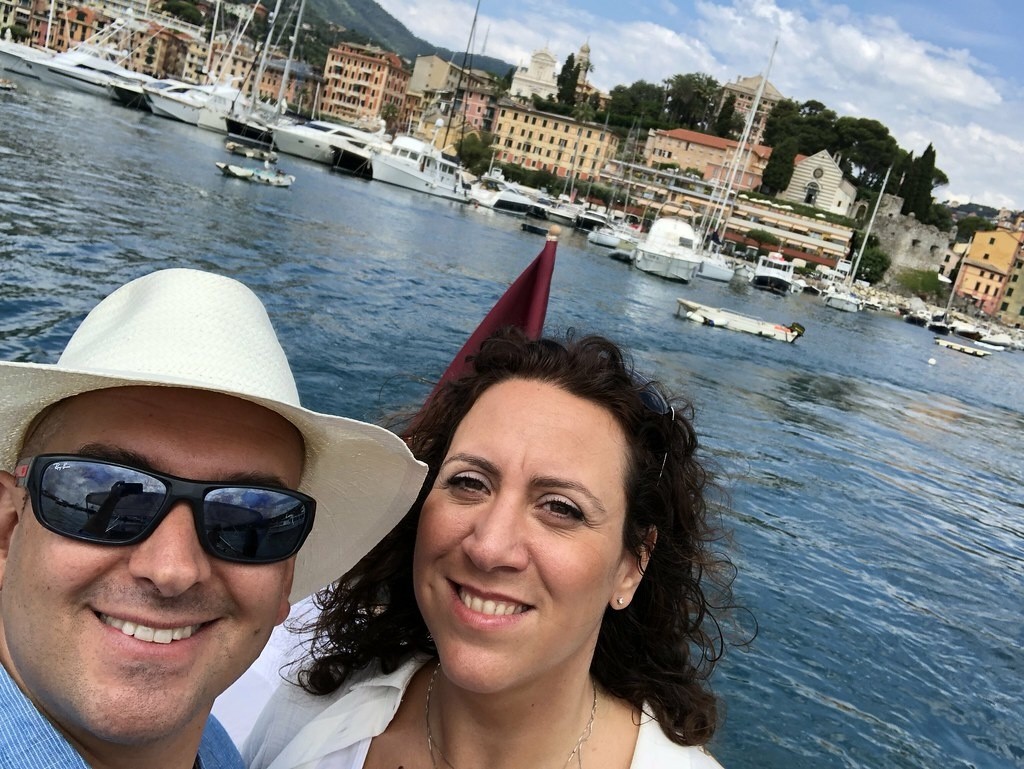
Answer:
(821, 167), (892, 314)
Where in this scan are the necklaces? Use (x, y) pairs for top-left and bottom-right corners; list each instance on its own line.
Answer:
(424, 659), (597, 769)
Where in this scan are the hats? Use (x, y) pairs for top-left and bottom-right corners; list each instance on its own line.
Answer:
(0, 267), (429, 606)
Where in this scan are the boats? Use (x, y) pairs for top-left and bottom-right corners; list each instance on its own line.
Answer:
(214, 161), (296, 188)
(225, 142), (279, 163)
(750, 240), (796, 298)
(933, 336), (993, 357)
(521, 223), (548, 236)
(674, 297), (806, 344)
(0, 0), (784, 285)
(790, 265), (1024, 353)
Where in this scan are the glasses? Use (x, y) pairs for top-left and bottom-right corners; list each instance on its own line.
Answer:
(610, 358), (676, 491)
(14, 453), (317, 565)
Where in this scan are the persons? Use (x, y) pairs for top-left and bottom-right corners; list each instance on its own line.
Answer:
(243, 326), (761, 769)
(0, 266), (430, 768)
